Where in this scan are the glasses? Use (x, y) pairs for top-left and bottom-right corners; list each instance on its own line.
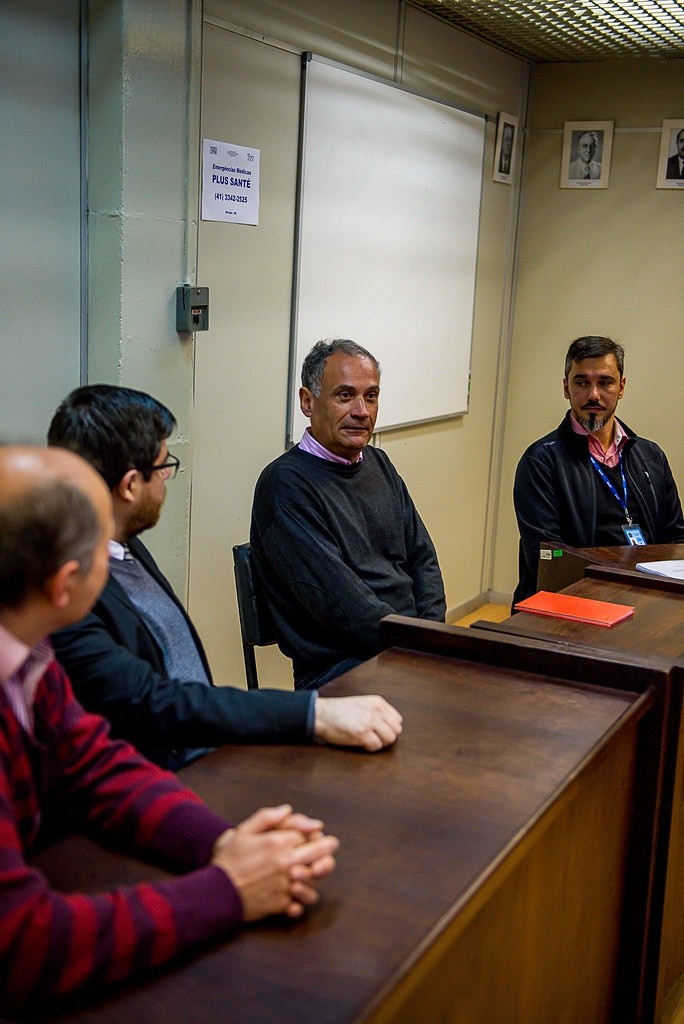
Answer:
(115, 454), (180, 489)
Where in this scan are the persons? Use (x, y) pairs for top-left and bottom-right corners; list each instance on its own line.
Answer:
(49, 385), (402, 773)
(513, 336), (684, 615)
(570, 131), (602, 180)
(250, 341), (446, 692)
(500, 126), (512, 174)
(667, 128), (684, 179)
(0, 446), (340, 1024)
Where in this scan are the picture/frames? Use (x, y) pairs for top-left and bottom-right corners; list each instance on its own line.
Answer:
(493, 112), (519, 186)
(657, 119), (684, 189)
(560, 121), (614, 189)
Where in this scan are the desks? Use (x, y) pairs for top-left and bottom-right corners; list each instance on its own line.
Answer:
(0, 543), (684, 1024)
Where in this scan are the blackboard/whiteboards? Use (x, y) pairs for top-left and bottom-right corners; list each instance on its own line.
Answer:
(285, 52), (489, 449)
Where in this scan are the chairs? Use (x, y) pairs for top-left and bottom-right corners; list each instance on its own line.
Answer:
(231, 542), (295, 692)
